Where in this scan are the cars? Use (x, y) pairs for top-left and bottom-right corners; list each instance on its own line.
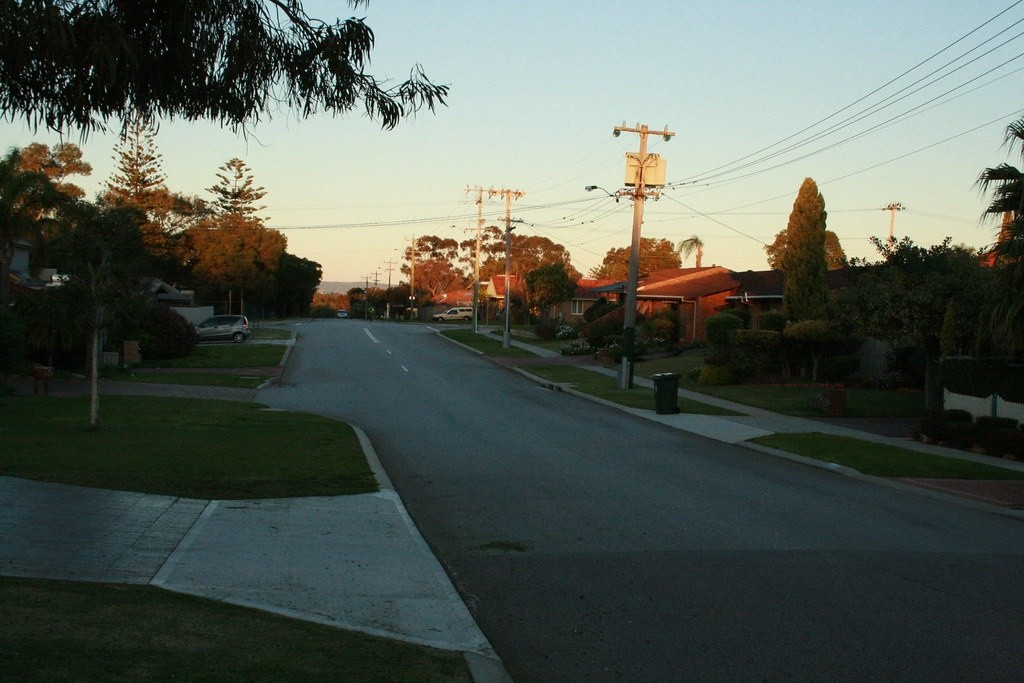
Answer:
(336, 309), (348, 319)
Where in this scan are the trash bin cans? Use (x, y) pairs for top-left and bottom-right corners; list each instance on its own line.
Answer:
(652, 372), (681, 414)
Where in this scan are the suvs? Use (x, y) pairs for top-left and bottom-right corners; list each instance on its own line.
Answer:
(433, 307), (474, 321)
(195, 314), (252, 344)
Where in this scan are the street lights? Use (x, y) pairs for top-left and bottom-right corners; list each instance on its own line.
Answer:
(448, 224), (479, 334)
(584, 185), (645, 390)
